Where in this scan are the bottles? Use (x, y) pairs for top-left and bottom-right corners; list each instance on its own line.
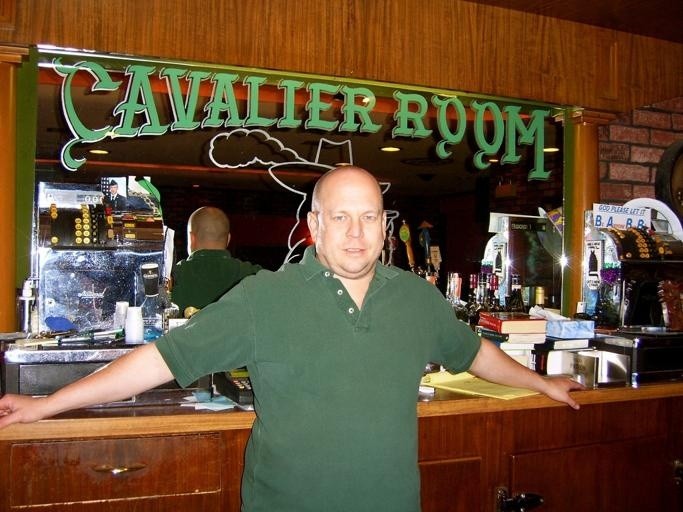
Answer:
(505, 272), (526, 312)
(494, 250), (503, 276)
(464, 272), (483, 326)
(483, 273), (502, 312)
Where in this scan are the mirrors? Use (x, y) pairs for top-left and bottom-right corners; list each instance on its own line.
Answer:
(29, 63), (565, 335)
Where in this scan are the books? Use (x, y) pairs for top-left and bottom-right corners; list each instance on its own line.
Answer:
(474, 311), (590, 376)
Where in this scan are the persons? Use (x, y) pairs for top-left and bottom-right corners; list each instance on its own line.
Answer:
(169, 203), (267, 315)
(0, 163), (589, 512)
(101, 180), (128, 210)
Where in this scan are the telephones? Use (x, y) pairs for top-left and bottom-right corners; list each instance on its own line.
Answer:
(213, 368), (255, 404)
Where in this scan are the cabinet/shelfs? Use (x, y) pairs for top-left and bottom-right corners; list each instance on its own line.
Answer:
(418, 396), (681, 509)
(1, 432), (227, 510)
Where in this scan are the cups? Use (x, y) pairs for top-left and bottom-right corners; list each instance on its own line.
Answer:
(140, 263), (160, 298)
(112, 301), (129, 329)
(125, 306), (143, 346)
